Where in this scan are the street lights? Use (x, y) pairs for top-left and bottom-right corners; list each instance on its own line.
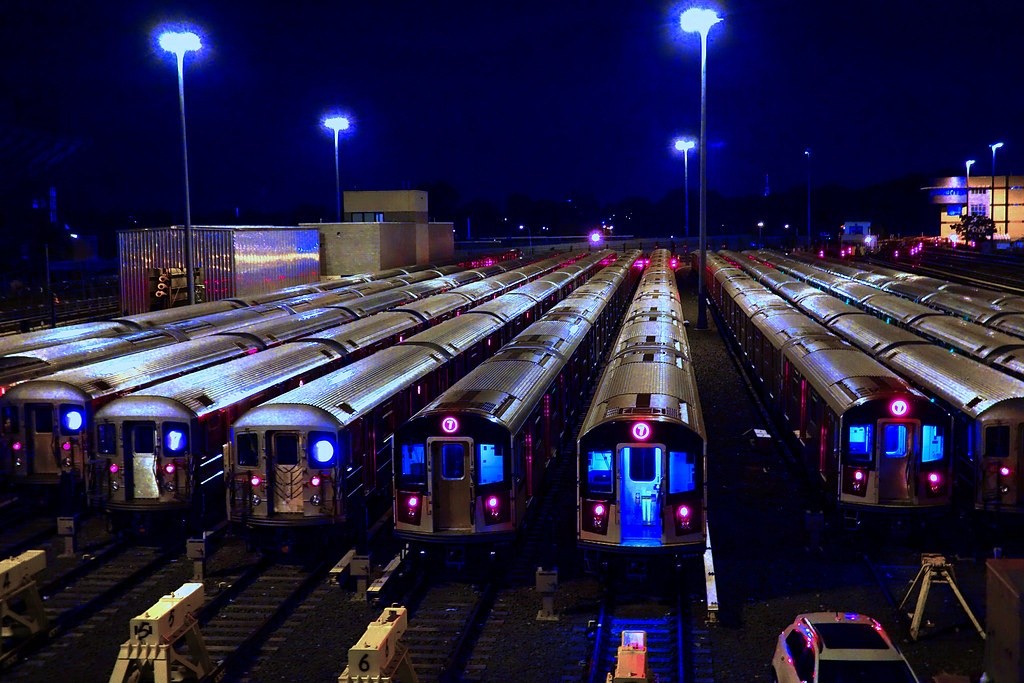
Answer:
(324, 116), (348, 223)
(679, 9), (722, 332)
(964, 160), (975, 253)
(676, 140), (696, 243)
(804, 152), (811, 248)
(158, 31), (202, 306)
(989, 141), (1004, 242)
(758, 222), (763, 245)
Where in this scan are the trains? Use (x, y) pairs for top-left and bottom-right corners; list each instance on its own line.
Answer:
(0, 248), (1024, 592)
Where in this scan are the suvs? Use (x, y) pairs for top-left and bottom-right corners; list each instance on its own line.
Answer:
(770, 610), (919, 682)
